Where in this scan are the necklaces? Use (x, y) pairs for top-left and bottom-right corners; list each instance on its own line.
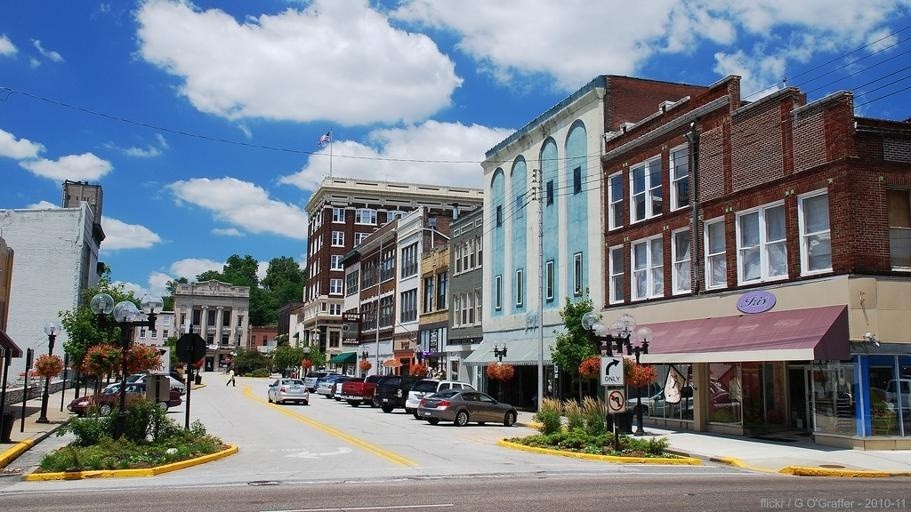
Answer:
(838, 381), (846, 395)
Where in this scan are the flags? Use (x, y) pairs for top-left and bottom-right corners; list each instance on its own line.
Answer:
(318, 130), (330, 145)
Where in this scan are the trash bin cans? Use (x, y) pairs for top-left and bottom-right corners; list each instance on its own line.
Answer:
(614, 410), (633, 433)
(3, 412), (16, 441)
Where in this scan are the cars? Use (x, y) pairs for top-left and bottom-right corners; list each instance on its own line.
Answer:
(629, 385), (695, 416)
(871, 377), (911, 415)
(68, 383), (182, 418)
(305, 370), (520, 426)
(104, 372), (186, 395)
(268, 375), (310, 406)
(169, 369), (186, 383)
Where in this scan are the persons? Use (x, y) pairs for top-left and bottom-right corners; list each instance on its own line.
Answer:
(290, 370), (297, 379)
(832, 375), (852, 432)
(226, 367), (236, 388)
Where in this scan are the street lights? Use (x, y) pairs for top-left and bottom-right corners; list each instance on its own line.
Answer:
(491, 340), (510, 400)
(91, 292), (164, 439)
(36, 323), (63, 424)
(580, 312), (637, 449)
(621, 325), (654, 436)
